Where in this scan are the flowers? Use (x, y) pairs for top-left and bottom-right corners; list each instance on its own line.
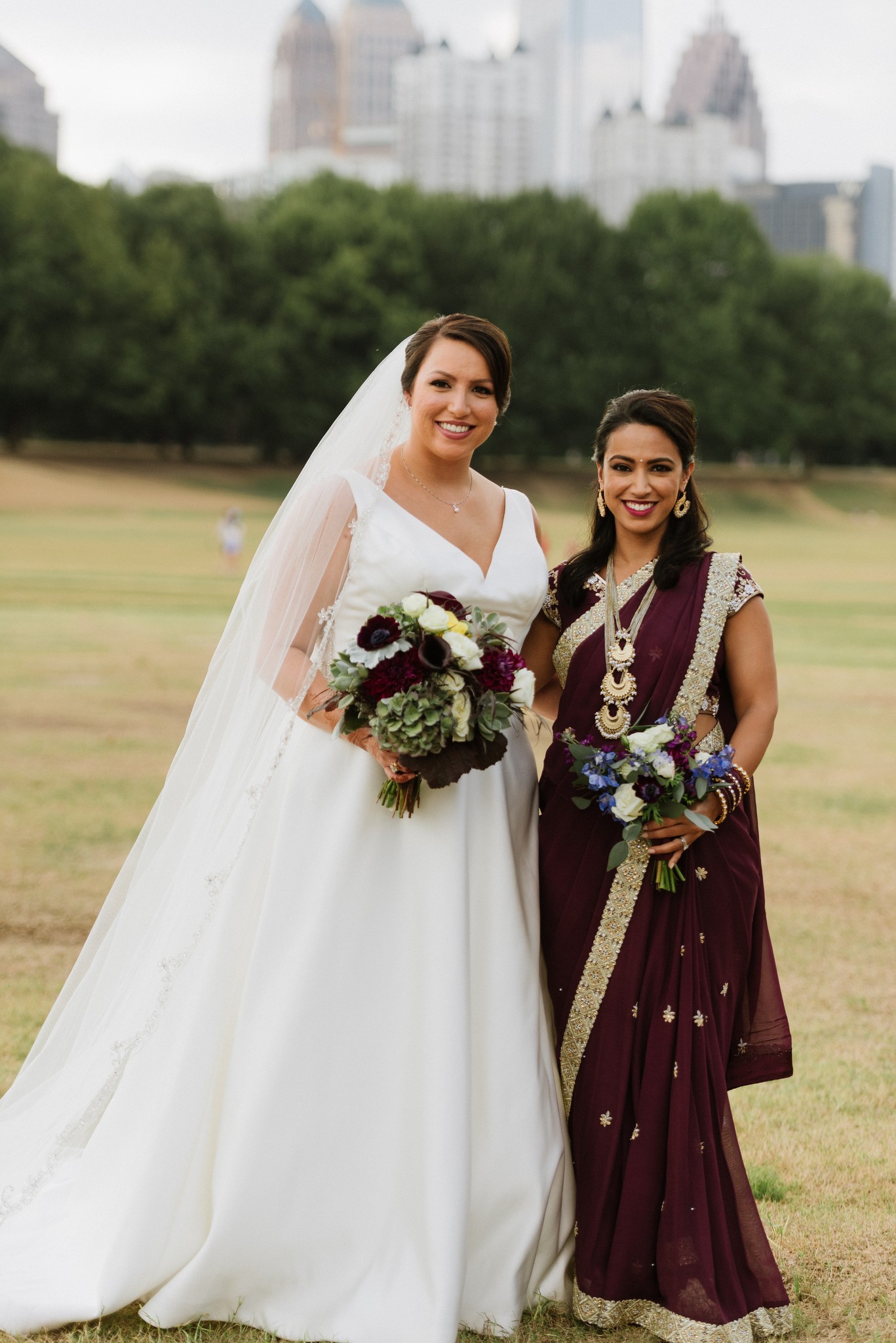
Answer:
(294, 586), (545, 822)
(557, 698), (737, 892)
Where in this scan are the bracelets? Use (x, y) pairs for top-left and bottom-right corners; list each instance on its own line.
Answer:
(731, 763), (751, 795)
(712, 778), (736, 813)
(709, 767), (745, 815)
(710, 784), (728, 824)
(725, 771), (741, 807)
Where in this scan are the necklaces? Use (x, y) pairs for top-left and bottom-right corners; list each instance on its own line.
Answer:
(401, 440), (472, 512)
(595, 554), (657, 739)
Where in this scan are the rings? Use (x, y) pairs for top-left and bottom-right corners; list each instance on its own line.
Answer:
(390, 759), (399, 773)
(680, 837), (688, 850)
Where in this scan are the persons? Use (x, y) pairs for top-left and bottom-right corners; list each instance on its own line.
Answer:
(515, 389), (796, 1343)
(0, 313), (581, 1343)
